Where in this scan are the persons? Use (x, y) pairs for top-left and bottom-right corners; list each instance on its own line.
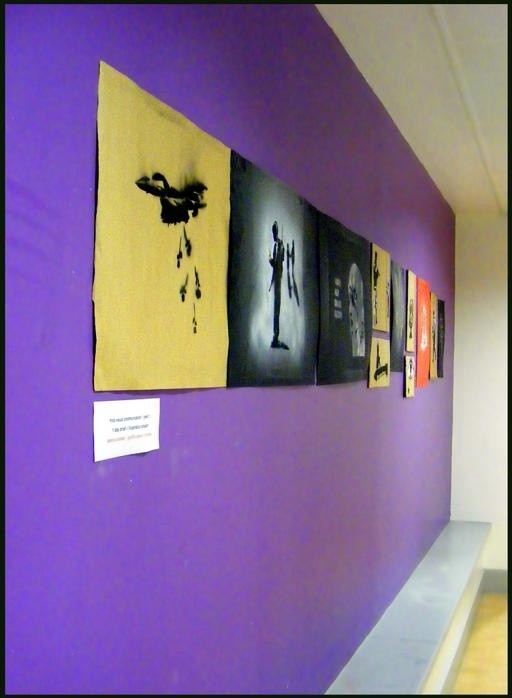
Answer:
(269, 219), (288, 349)
(372, 250), (380, 327)
